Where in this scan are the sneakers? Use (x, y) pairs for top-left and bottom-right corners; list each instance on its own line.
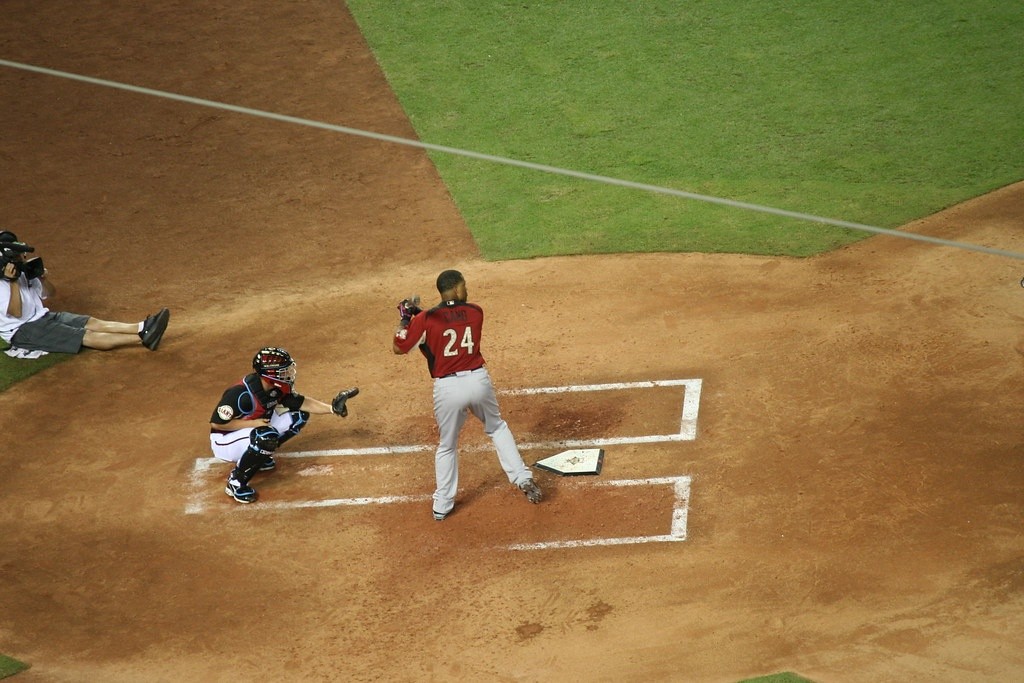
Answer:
(257, 455), (276, 470)
(433, 510), (445, 519)
(225, 468), (256, 502)
(148, 322), (168, 351)
(138, 306), (168, 347)
(519, 478), (542, 503)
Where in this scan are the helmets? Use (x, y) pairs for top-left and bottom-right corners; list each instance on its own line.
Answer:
(253, 346), (297, 385)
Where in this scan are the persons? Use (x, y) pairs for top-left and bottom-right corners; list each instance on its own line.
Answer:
(209, 347), (359, 505)
(392, 269), (545, 521)
(0, 230), (170, 355)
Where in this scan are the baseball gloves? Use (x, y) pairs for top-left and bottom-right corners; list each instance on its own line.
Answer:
(332, 387), (359, 417)
